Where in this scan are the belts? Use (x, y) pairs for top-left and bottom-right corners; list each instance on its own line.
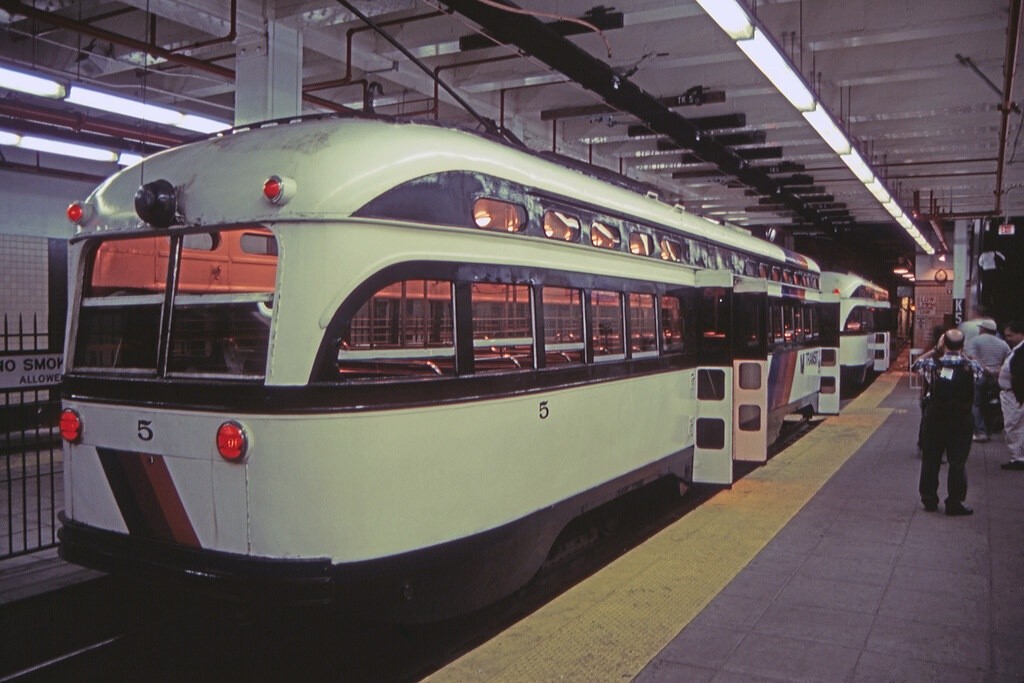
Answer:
(1001, 388), (1012, 391)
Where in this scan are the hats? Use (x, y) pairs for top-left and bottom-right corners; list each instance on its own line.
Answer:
(976, 320), (997, 330)
(973, 305), (986, 315)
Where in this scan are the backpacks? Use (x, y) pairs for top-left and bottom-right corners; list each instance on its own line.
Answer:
(929, 356), (971, 422)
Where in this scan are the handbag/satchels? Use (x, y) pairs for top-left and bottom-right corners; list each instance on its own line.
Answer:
(993, 250), (1006, 271)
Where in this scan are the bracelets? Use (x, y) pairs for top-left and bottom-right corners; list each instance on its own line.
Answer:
(934, 345), (940, 352)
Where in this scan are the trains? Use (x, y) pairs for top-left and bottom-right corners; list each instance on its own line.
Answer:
(56, 112), (890, 618)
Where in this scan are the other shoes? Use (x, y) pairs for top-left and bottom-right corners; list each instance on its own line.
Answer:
(1001, 460), (1024, 470)
(925, 506), (937, 512)
(972, 432), (988, 441)
(946, 505), (974, 516)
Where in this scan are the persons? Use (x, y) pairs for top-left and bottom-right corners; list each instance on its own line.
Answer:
(978, 244), (1006, 294)
(956, 303), (1013, 441)
(999, 322), (1024, 470)
(910, 329), (983, 515)
